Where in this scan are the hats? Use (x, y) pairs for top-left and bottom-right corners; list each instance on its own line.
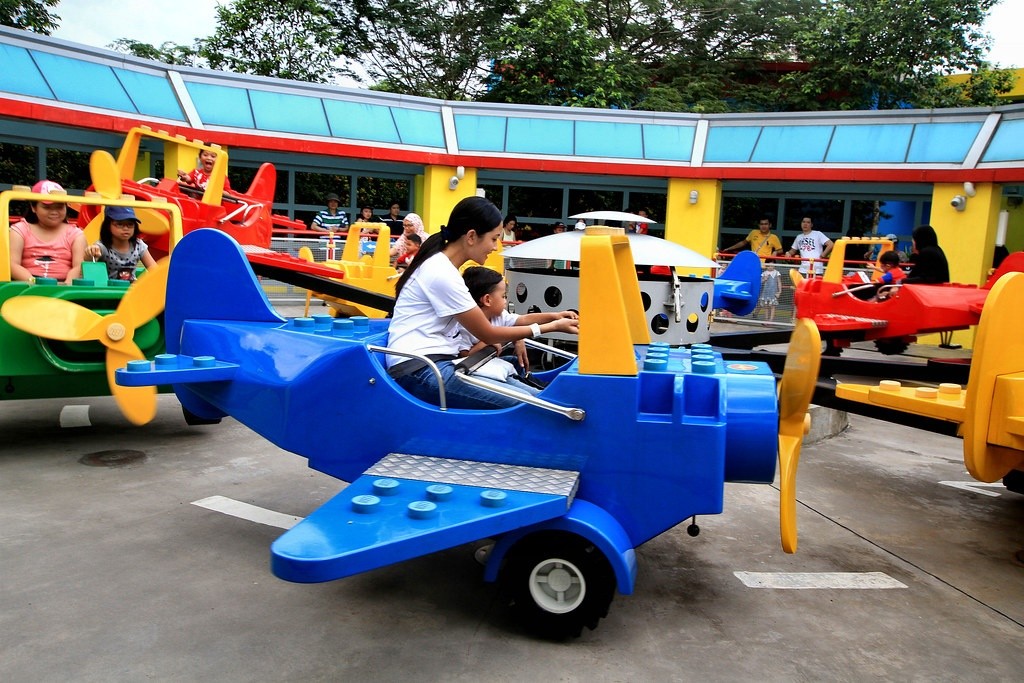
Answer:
(105, 205), (141, 223)
(326, 193), (341, 201)
(554, 221), (567, 228)
(33, 179), (67, 204)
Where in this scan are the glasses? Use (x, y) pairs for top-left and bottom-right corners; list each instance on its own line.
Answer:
(111, 221), (135, 227)
(403, 224), (413, 227)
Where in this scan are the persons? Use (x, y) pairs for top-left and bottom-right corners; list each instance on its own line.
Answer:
(896, 225), (950, 284)
(573, 219), (587, 231)
(354, 203), (379, 257)
(498, 213), (522, 269)
(545, 222), (571, 269)
(720, 216), (784, 322)
(622, 208), (649, 235)
(385, 194), (579, 410)
(311, 193), (348, 261)
(784, 214), (834, 279)
(390, 213), (431, 257)
(176, 142), (232, 195)
(376, 200), (405, 241)
(392, 234), (422, 273)
(864, 233), (908, 263)
(866, 251), (907, 292)
(9, 179), (85, 286)
(83, 206), (158, 283)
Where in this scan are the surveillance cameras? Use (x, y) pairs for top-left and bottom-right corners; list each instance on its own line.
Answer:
(951, 197), (961, 207)
(450, 176), (459, 186)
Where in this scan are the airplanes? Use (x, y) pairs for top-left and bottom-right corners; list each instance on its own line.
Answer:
(0, 149), (226, 428)
(112, 226), (822, 644)
(82, 125), (1024, 511)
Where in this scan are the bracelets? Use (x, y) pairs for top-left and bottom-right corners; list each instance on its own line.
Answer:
(529, 322), (541, 339)
(820, 254), (825, 259)
